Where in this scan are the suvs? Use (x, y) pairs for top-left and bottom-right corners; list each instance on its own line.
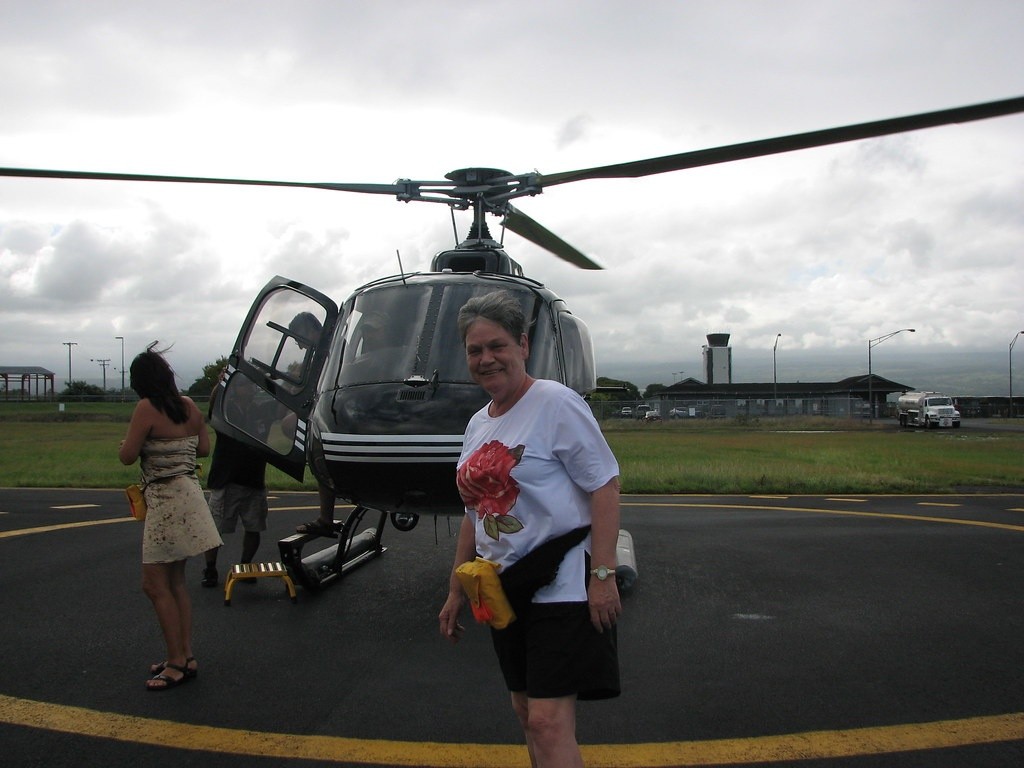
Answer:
(620, 405), (727, 419)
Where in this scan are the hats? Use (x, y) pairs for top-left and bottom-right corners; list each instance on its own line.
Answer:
(360, 309), (394, 329)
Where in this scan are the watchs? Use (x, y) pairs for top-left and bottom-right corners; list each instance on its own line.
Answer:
(591, 565), (616, 581)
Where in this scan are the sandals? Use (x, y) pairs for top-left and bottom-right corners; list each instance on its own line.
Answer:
(296, 518), (338, 539)
(145, 661), (188, 691)
(150, 656), (197, 675)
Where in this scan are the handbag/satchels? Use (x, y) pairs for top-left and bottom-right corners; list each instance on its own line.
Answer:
(454, 561), (516, 628)
(125, 484), (148, 521)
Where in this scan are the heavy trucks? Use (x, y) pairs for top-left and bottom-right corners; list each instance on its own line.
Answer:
(897, 391), (962, 430)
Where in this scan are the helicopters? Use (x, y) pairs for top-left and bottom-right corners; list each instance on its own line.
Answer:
(1, 96), (1024, 596)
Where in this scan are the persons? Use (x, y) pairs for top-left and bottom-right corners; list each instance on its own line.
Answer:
(202, 368), (298, 587)
(440, 290), (623, 768)
(337, 311), (410, 415)
(282, 312), (348, 538)
(119, 342), (224, 690)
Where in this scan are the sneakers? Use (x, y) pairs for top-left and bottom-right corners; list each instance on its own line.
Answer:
(201, 567), (218, 587)
(239, 566), (258, 584)
